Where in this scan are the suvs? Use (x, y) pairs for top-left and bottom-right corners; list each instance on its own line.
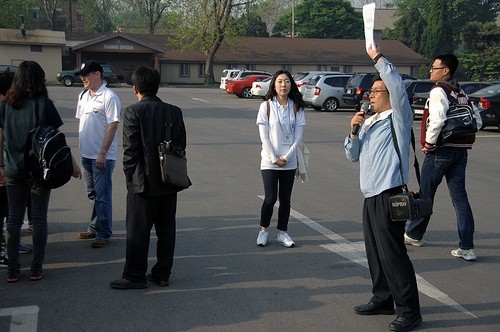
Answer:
(411, 78), (437, 116)
(302, 74), (356, 112)
(341, 72), (418, 114)
(219, 69), (240, 90)
(293, 70), (345, 97)
(231, 69), (272, 81)
(402, 79), (419, 106)
(56, 64), (119, 88)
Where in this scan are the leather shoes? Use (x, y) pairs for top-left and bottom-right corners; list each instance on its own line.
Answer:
(389, 316), (423, 331)
(353, 302), (396, 315)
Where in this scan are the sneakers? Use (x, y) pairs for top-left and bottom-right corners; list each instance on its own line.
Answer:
(277, 232), (296, 248)
(256, 230), (269, 246)
(0, 243), (9, 267)
(451, 248), (477, 260)
(404, 233), (424, 247)
(17, 242), (32, 253)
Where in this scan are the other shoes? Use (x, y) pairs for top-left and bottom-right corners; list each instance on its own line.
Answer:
(147, 273), (169, 286)
(90, 238), (107, 247)
(30, 269), (45, 280)
(7, 271), (19, 281)
(28, 225), (34, 232)
(78, 231), (93, 238)
(111, 278), (147, 289)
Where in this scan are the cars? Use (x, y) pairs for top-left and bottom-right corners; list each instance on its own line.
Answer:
(224, 75), (271, 99)
(251, 74), (295, 101)
(468, 84), (500, 131)
(0, 64), (18, 96)
(458, 81), (494, 95)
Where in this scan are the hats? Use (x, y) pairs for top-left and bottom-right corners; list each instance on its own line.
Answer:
(74, 59), (101, 75)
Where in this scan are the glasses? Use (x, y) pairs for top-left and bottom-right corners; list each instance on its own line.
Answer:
(368, 89), (389, 98)
(431, 65), (448, 73)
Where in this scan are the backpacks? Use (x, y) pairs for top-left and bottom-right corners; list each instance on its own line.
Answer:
(28, 100), (73, 189)
(435, 83), (478, 146)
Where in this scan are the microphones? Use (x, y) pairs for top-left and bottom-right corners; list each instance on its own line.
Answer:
(352, 102), (369, 134)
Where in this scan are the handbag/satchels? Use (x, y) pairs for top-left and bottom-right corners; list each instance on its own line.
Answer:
(388, 191), (424, 221)
(158, 143), (189, 190)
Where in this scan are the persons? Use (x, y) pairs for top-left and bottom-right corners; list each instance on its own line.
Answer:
(343, 44), (423, 332)
(404, 54), (483, 259)
(110, 66), (186, 290)
(73, 61), (121, 248)
(257, 69), (306, 248)
(0, 61), (82, 281)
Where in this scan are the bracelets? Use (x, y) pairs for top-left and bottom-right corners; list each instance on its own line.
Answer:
(373, 54), (383, 64)
